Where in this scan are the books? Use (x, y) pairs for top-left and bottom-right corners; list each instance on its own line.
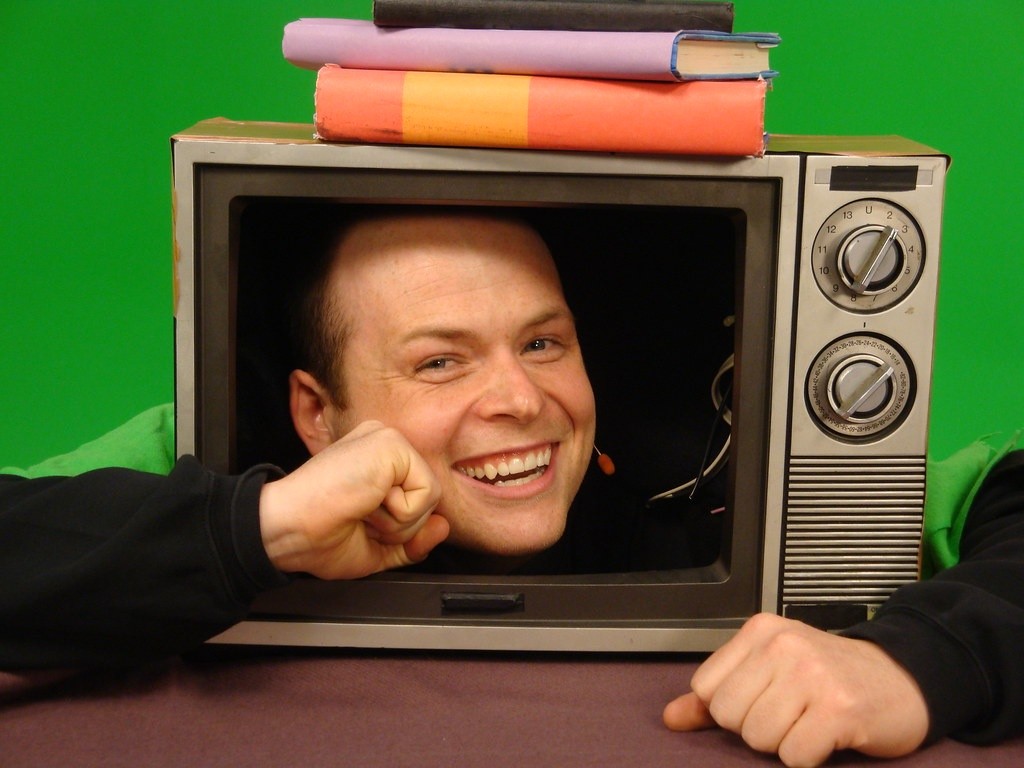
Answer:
(371, 0), (735, 34)
(282, 17), (780, 82)
(312, 65), (769, 157)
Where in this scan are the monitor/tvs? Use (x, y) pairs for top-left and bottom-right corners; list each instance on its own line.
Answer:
(175, 115), (945, 654)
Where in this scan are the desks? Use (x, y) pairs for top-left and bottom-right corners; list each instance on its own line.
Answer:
(0, 613), (1024, 768)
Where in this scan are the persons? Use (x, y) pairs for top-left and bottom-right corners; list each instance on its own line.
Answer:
(2, 210), (1024, 768)
